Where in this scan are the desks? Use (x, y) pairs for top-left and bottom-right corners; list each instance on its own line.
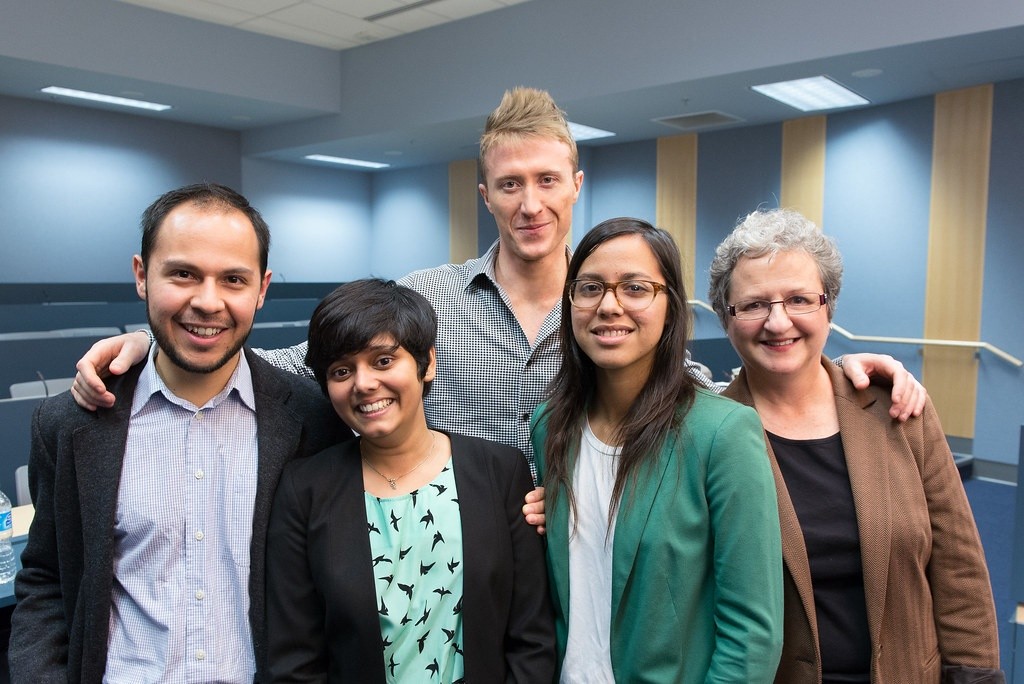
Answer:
(0, 536), (31, 606)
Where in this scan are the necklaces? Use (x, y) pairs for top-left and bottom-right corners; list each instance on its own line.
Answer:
(357, 428), (436, 492)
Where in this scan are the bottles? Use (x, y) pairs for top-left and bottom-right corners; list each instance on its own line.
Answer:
(0, 490), (17, 585)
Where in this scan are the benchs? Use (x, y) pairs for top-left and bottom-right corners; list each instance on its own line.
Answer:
(1, 399), (38, 506)
(1, 299), (328, 400)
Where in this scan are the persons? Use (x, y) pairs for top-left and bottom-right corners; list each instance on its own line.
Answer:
(0, 182), (549, 683)
(254, 275), (562, 683)
(705, 205), (1006, 684)
(68, 81), (932, 499)
(521, 216), (793, 683)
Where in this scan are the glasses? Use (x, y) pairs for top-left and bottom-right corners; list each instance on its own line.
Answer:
(724, 292), (828, 321)
(565, 278), (670, 312)
